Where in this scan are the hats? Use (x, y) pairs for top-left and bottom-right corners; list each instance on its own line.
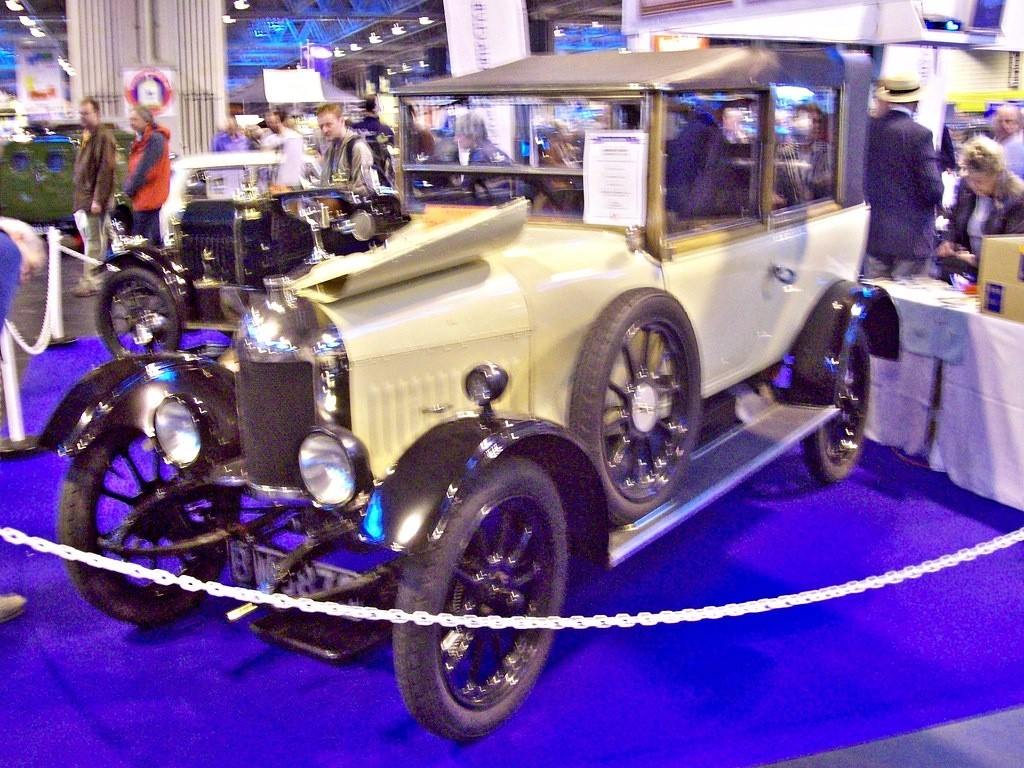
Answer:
(875, 75), (924, 102)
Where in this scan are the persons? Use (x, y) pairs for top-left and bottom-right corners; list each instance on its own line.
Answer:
(247, 96), (420, 255)
(666, 99), (833, 217)
(863, 76), (1024, 362)
(70, 97), (117, 296)
(410, 103), (514, 191)
(214, 113), (251, 153)
(120, 105), (171, 247)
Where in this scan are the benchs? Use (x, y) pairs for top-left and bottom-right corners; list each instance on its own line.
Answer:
(663, 152), (813, 204)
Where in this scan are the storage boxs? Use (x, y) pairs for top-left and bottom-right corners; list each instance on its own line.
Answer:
(977, 234), (1023, 322)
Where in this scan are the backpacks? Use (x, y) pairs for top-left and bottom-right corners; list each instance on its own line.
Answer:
(345, 136), (394, 188)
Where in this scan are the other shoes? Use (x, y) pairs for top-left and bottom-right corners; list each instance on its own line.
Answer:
(0, 595), (27, 622)
(69, 280), (105, 296)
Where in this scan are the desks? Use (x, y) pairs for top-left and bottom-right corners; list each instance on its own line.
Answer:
(859, 276), (1024, 513)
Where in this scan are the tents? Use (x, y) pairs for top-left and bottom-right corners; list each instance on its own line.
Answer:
(229, 68), (368, 115)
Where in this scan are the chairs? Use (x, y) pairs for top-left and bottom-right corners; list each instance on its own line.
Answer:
(665, 113), (740, 223)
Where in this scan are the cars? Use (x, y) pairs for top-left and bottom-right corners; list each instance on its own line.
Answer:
(90, 186), (413, 362)
(19, 121), (209, 197)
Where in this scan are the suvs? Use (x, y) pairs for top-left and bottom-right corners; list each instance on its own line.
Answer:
(35, 49), (905, 746)
